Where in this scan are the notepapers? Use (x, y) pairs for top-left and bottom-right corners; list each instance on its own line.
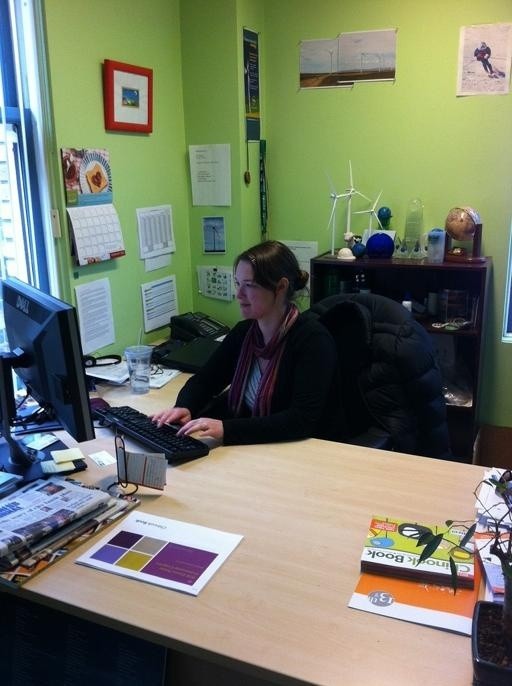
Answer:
(50, 447), (85, 464)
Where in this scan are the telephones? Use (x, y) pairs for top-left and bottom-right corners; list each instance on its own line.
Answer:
(170, 311), (231, 340)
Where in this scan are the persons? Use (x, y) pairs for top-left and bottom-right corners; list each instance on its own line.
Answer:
(148, 240), (338, 446)
(474, 42), (495, 75)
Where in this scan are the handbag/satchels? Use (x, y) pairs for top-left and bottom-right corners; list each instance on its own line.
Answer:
(162, 336), (222, 372)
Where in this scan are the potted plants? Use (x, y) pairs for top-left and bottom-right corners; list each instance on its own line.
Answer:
(471, 469), (511, 686)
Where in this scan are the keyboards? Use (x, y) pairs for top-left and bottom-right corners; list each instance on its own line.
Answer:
(94, 406), (209, 464)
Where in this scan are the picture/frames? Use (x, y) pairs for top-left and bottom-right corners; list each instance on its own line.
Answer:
(104, 59), (153, 133)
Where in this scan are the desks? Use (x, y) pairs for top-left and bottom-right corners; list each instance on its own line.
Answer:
(0, 335), (510, 686)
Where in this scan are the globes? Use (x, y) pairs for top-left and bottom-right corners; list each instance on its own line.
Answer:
(444, 206), (486, 264)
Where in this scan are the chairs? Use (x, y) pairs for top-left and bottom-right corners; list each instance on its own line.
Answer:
(300, 294), (454, 463)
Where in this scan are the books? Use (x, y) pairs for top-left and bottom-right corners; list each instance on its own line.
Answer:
(359, 512), (477, 585)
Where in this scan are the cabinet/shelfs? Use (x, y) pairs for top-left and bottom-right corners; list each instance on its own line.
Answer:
(309, 248), (490, 433)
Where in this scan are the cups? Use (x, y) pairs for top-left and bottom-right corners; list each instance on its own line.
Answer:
(421, 231), (446, 263)
(125, 345), (154, 394)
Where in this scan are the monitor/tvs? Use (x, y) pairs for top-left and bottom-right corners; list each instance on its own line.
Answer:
(0, 276), (96, 483)
